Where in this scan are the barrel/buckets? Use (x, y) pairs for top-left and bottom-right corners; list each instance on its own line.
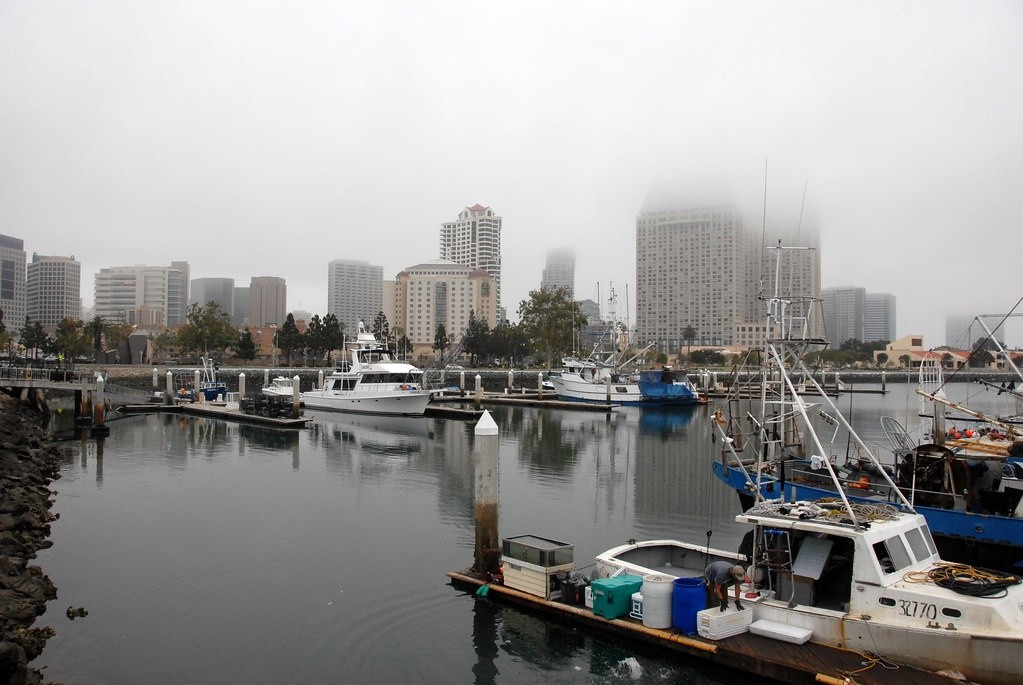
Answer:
(671, 576), (708, 635)
(641, 573), (674, 628)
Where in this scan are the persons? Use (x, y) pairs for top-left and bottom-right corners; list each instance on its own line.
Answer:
(704, 561), (746, 612)
(56, 352), (64, 369)
(180, 386), (186, 404)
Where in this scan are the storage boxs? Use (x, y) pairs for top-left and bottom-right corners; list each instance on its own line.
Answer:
(643, 574), (675, 629)
(697, 602), (753, 640)
(501, 556), (576, 600)
(672, 577), (706, 636)
(585, 586), (593, 609)
(749, 618), (812, 645)
(630, 592), (644, 620)
(501, 534), (574, 568)
(591, 575), (643, 620)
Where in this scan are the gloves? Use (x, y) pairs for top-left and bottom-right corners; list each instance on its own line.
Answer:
(735, 600), (744, 611)
(719, 598), (728, 613)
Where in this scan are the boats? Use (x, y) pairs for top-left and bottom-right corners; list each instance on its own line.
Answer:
(304, 322), (431, 415)
(544, 281), (714, 408)
(176, 356), (228, 401)
(921, 291), (1023, 461)
(701, 291), (1023, 551)
(260, 331), (304, 405)
(445, 240), (1023, 684)
(423, 399), (493, 419)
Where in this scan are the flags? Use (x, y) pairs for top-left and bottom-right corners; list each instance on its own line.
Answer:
(997, 379), (1015, 395)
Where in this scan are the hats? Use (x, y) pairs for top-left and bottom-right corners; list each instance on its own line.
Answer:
(733, 566), (745, 580)
